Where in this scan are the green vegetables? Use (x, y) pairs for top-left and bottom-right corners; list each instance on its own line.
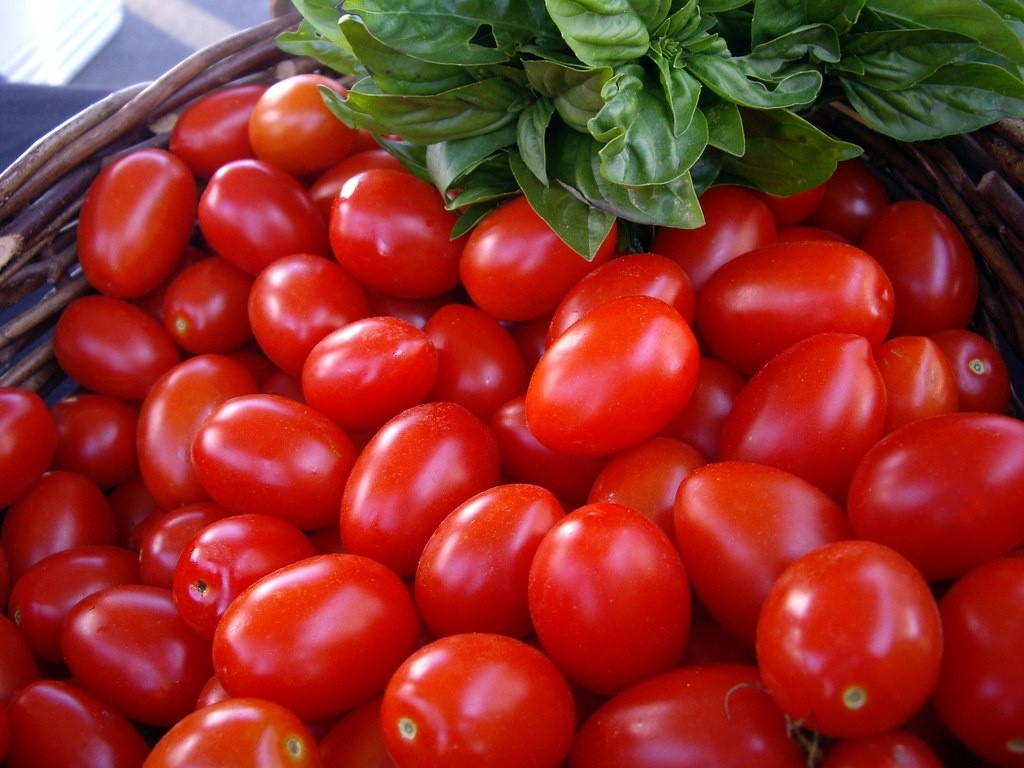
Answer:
(274, 0), (1024, 262)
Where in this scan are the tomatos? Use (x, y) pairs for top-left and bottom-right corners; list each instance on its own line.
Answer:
(0, 74), (1024, 768)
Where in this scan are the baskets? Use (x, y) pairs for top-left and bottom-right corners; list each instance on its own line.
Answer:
(0, 0), (1024, 431)
